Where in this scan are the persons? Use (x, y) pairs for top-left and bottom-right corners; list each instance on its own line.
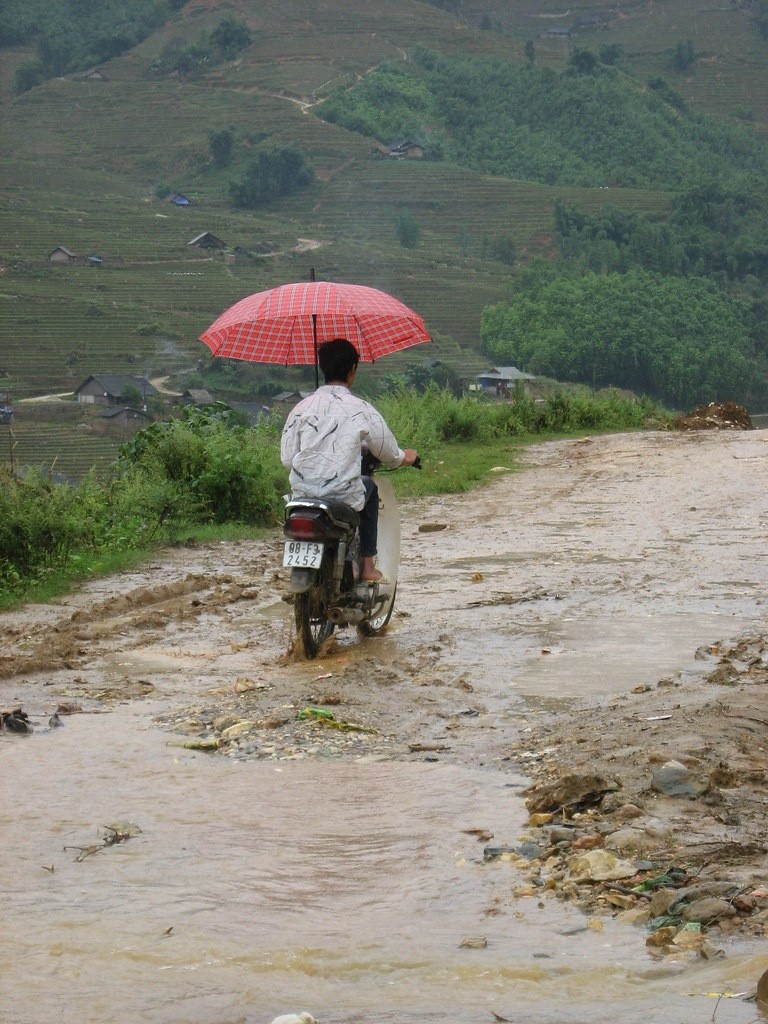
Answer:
(280, 338), (420, 583)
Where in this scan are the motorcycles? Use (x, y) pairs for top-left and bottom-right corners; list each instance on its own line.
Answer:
(280, 439), (425, 660)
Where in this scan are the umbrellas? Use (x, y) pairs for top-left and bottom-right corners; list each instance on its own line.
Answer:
(196, 268), (434, 391)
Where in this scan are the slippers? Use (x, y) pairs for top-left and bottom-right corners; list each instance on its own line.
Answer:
(365, 570), (391, 584)
